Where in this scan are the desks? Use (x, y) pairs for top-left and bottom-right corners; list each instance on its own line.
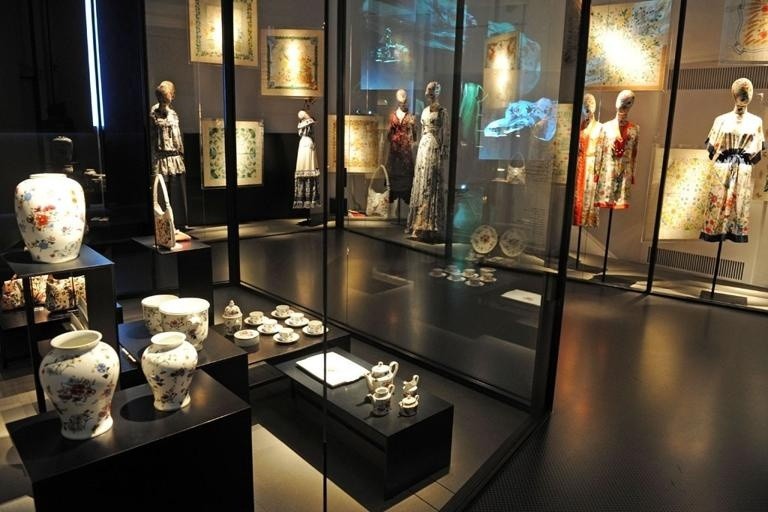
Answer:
(210, 304), (350, 395)
(110, 314), (248, 403)
(6, 365), (255, 512)
(263, 343), (456, 506)
(0, 300), (73, 358)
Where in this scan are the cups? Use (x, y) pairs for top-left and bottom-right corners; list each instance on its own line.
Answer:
(483, 273), (494, 280)
(262, 320), (277, 332)
(469, 277), (480, 285)
(433, 269), (443, 275)
(451, 272), (462, 280)
(479, 268), (496, 280)
(447, 265), (458, 272)
(249, 311), (263, 323)
(276, 305), (289, 316)
(279, 328), (294, 340)
(291, 313), (304, 324)
(464, 269), (476, 276)
(308, 320), (322, 333)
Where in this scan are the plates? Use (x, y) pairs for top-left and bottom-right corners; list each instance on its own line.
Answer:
(500, 227), (528, 258)
(296, 351), (370, 387)
(444, 268), (461, 275)
(302, 326), (329, 335)
(429, 272), (447, 278)
(470, 224), (498, 254)
(284, 319), (308, 326)
(464, 281), (485, 287)
(243, 316), (270, 326)
(273, 333), (300, 344)
(271, 310), (295, 318)
(461, 273), (479, 278)
(447, 276), (466, 282)
(479, 276), (497, 282)
(257, 324), (283, 334)
(234, 329), (260, 346)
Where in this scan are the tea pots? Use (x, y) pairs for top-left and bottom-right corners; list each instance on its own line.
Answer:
(398, 394), (419, 416)
(365, 383), (396, 415)
(362, 360), (400, 393)
(402, 374), (419, 397)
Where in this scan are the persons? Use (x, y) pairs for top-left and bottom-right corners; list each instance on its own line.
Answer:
(700, 77), (768, 243)
(571, 93), (606, 227)
(595, 90), (641, 211)
(151, 79), (195, 231)
(291, 109), (322, 226)
(384, 87), (420, 206)
(403, 80), (449, 240)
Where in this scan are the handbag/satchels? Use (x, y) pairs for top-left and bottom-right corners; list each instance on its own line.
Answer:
(506, 152), (527, 185)
(153, 170), (176, 249)
(366, 164), (391, 217)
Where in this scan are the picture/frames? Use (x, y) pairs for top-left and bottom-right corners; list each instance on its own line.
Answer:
(257, 24), (327, 101)
(323, 110), (390, 176)
(582, 0), (678, 92)
(199, 117), (264, 190)
(186, 0), (260, 68)
(715, 0), (768, 64)
(478, 29), (521, 111)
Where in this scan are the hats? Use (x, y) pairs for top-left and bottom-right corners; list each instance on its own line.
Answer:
(295, 110), (316, 130)
(484, 96), (552, 137)
